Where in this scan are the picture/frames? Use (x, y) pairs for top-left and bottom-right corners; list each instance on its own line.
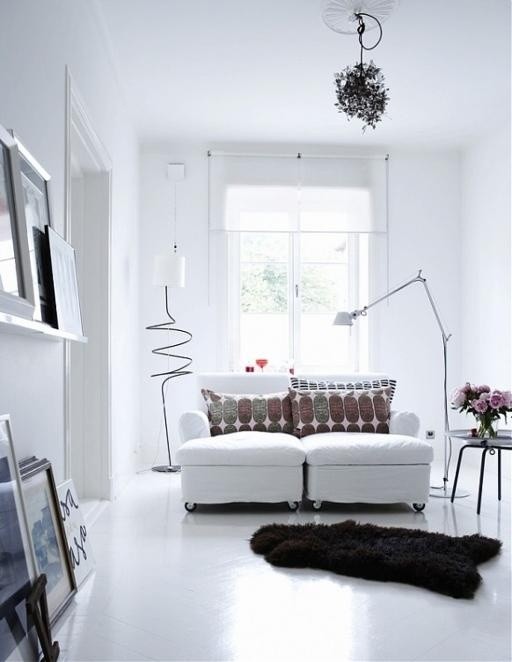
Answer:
(0, 414), (79, 662)
(0, 122), (84, 337)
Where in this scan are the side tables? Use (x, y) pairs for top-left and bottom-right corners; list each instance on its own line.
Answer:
(440, 429), (512, 514)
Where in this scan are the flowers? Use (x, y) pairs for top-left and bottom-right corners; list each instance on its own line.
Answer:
(330, 61), (388, 136)
(450, 378), (512, 438)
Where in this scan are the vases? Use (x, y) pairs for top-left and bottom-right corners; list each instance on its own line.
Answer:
(473, 414), (500, 437)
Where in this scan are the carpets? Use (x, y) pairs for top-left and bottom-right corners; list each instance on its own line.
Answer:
(251, 518), (502, 599)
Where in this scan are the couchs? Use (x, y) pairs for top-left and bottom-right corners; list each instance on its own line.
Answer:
(174, 370), (433, 512)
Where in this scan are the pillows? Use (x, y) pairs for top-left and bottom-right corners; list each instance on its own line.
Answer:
(201, 376), (398, 436)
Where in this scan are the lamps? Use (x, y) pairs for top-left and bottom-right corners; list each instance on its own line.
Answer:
(146, 253), (196, 473)
(330, 267), (472, 499)
